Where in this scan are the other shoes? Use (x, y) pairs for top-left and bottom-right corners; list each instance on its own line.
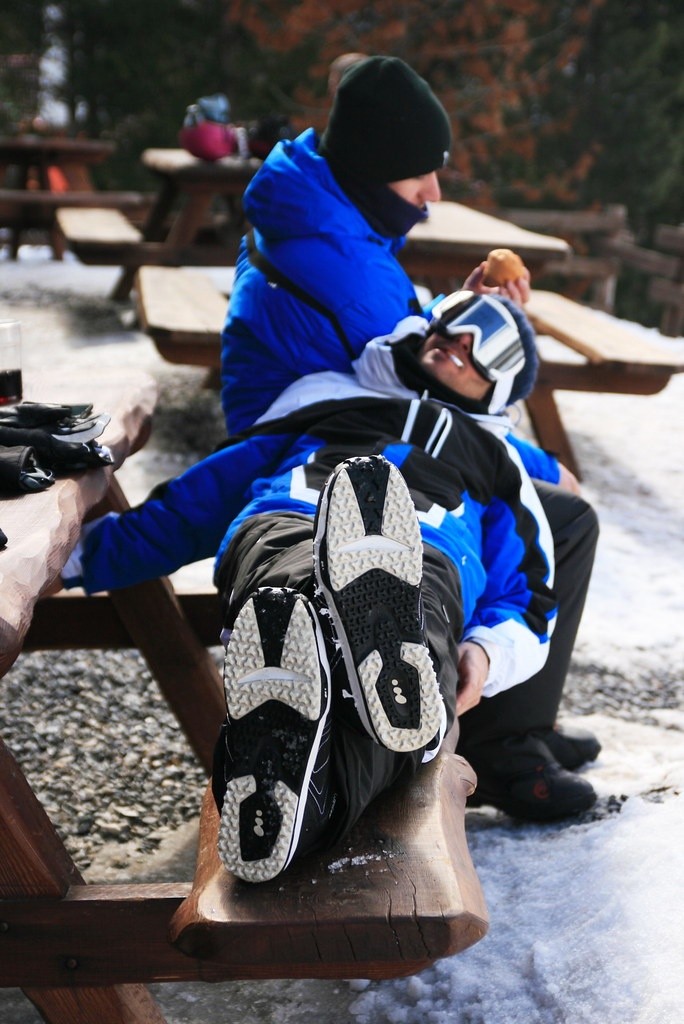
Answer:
(313, 456), (439, 751)
(219, 588), (334, 881)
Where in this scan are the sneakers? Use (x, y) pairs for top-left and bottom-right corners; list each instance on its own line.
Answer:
(451, 730), (600, 825)
(528, 721), (603, 773)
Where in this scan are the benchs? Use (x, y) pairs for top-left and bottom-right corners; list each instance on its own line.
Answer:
(0, 186), (235, 365)
(166, 718), (489, 967)
(518, 287), (683, 397)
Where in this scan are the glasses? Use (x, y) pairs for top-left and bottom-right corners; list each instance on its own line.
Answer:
(434, 294), (525, 386)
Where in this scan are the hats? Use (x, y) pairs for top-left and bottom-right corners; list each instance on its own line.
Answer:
(317, 58), (452, 190)
(429, 294), (540, 405)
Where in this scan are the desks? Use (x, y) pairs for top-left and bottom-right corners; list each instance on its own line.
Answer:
(0, 363), (227, 1024)
(407, 200), (583, 483)
(109, 150), (266, 302)
(0, 131), (114, 264)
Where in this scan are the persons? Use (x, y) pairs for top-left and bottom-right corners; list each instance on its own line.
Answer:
(52, 289), (559, 883)
(217, 55), (598, 825)
